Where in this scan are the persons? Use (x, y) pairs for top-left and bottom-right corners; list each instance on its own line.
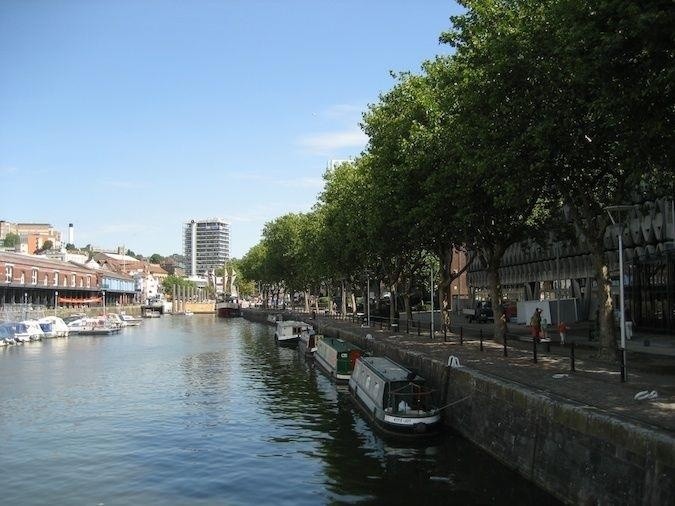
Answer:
(559, 320), (566, 345)
(443, 308), (452, 333)
(530, 308), (542, 339)
(624, 304), (636, 340)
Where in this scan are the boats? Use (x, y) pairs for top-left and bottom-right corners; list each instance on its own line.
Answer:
(291, 352), (314, 381)
(0, 311), (142, 348)
(348, 354), (441, 433)
(274, 342), (299, 369)
(0, 324), (139, 360)
(298, 328), (329, 359)
(141, 308), (161, 319)
(167, 310), (193, 316)
(314, 336), (365, 382)
(310, 356), (351, 416)
(346, 390), (439, 465)
(274, 320), (313, 348)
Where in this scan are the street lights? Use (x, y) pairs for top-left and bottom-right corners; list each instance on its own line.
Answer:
(220, 276), (347, 322)
(361, 266), (374, 325)
(603, 204), (636, 383)
(425, 253), (437, 339)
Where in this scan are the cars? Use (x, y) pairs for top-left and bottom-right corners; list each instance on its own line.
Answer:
(502, 298), (519, 325)
(468, 301), (494, 322)
